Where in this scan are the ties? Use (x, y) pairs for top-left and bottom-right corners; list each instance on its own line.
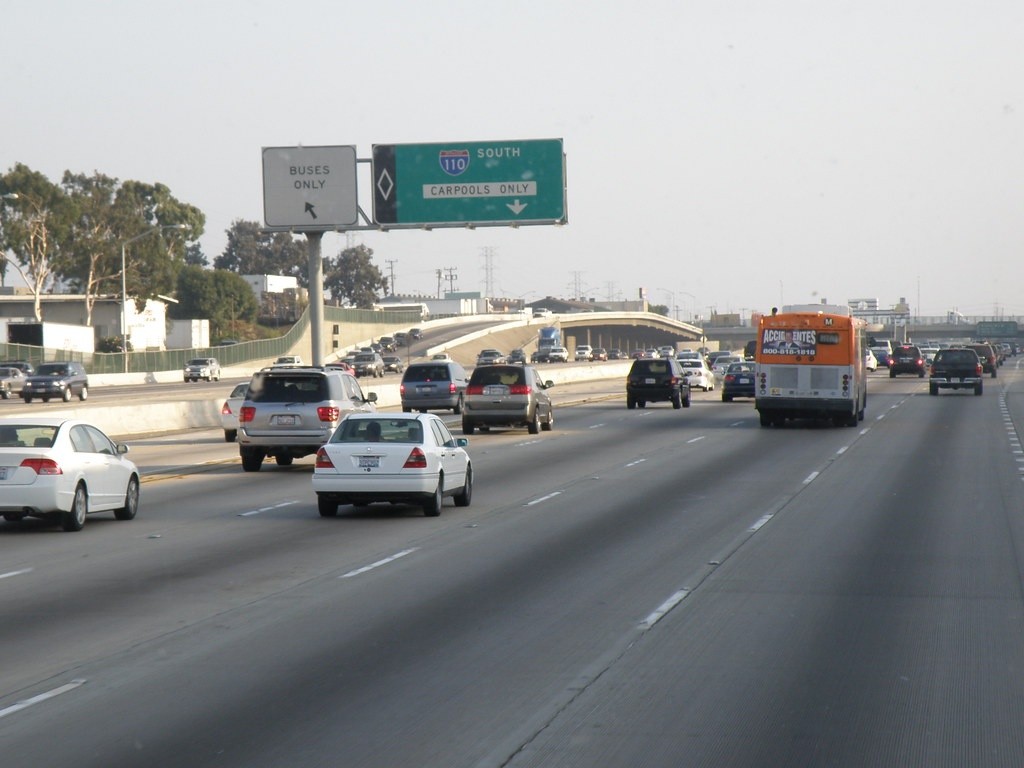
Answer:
(786, 344), (788, 349)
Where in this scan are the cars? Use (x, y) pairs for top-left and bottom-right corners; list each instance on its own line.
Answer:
(866, 336), (1020, 379)
(0, 419), (140, 532)
(476, 345), (757, 402)
(222, 382), (252, 441)
(325, 327), (423, 380)
(463, 364), (554, 435)
(533, 308), (551, 316)
(312, 410), (472, 517)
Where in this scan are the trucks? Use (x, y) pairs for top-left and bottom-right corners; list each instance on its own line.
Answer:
(755, 304), (874, 427)
(535, 328), (560, 350)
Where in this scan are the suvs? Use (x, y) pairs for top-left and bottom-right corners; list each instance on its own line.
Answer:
(627, 355), (690, 410)
(238, 366), (379, 472)
(350, 352), (385, 378)
(889, 342), (983, 394)
(0, 358), (88, 404)
(184, 358), (221, 383)
(274, 356), (304, 365)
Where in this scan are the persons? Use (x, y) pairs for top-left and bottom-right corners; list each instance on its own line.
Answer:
(777, 331), (800, 354)
(367, 422), (380, 442)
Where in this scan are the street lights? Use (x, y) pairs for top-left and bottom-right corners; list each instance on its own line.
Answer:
(122, 224), (185, 373)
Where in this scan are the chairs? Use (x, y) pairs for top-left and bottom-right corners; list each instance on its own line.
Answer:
(0, 438), (52, 449)
(358, 426), (423, 443)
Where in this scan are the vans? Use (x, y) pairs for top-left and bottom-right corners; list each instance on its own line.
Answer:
(399, 359), (471, 416)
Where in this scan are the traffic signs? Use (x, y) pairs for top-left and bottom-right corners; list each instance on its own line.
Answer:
(262, 138), (565, 227)
